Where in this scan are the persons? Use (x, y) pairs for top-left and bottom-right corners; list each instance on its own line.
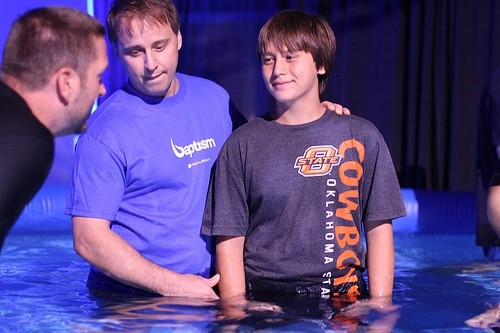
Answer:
(464, 304), (500, 328)
(200, 9), (408, 307)
(63, 0), (352, 301)
(215, 307), (400, 332)
(72, 301), (220, 333)
(1, 5), (109, 253)
(476, 143), (500, 261)
